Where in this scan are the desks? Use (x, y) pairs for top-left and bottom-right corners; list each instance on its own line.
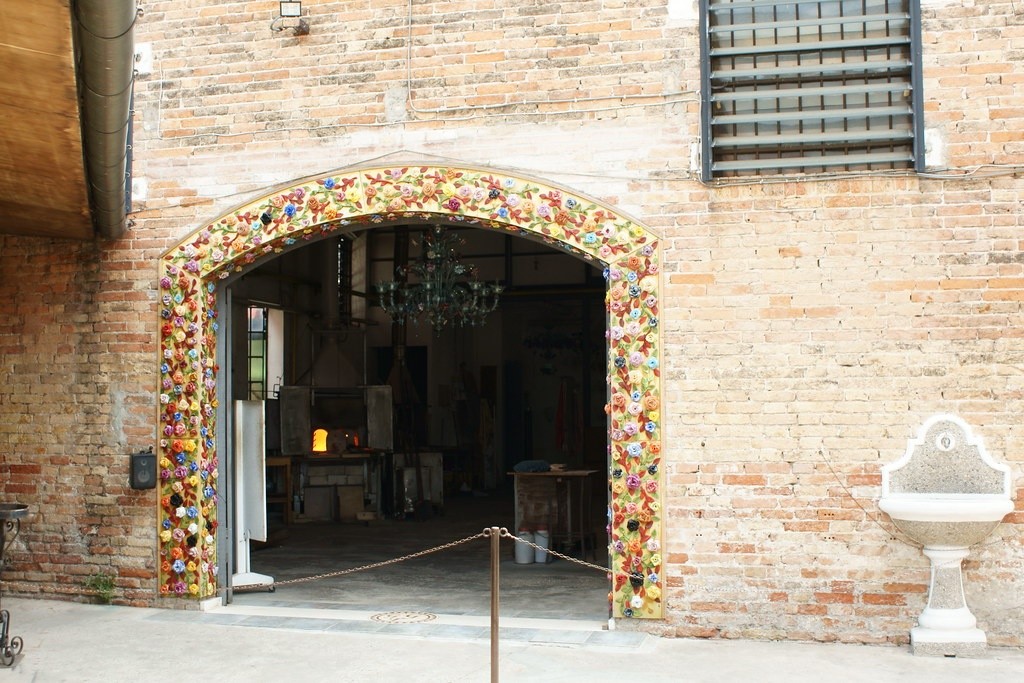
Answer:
(0, 503), (30, 666)
(509, 471), (597, 565)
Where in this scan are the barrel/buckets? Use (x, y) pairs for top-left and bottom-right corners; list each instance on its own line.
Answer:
(514, 524), (553, 563)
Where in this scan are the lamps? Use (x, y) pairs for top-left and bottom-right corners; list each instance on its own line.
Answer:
(378, 222), (500, 339)
(270, 0), (309, 37)
